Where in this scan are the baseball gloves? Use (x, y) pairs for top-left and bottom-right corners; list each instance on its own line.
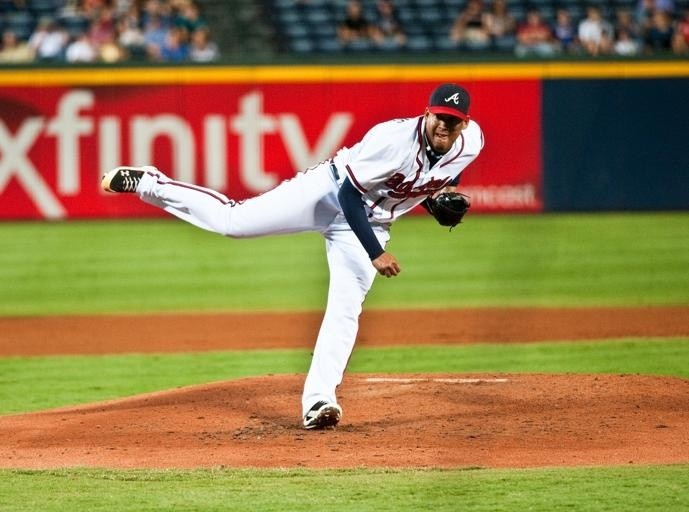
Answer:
(431, 192), (471, 231)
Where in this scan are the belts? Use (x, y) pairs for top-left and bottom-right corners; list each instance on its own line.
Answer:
(330, 158), (340, 180)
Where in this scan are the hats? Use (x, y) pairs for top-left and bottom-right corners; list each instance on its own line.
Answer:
(428, 84), (470, 121)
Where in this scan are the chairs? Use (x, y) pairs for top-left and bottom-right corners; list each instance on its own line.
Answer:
(1, 0), (689, 57)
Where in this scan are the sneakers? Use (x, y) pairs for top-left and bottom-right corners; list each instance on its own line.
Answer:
(101, 166), (158, 193)
(304, 401), (342, 429)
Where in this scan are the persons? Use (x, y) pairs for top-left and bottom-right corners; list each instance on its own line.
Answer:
(0, 0), (689, 63)
(102, 82), (484, 430)
